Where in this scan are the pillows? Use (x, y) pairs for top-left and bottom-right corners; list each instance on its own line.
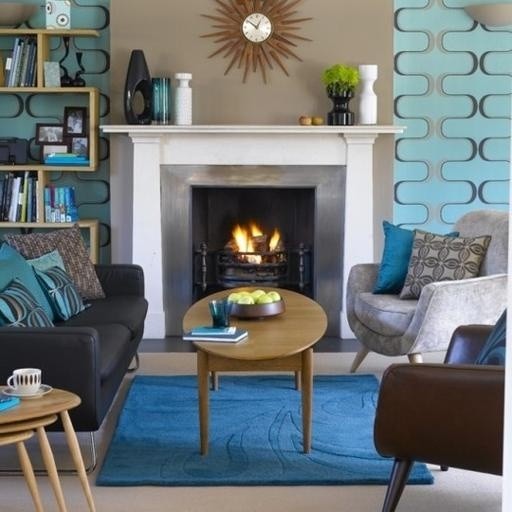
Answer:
(372, 217), (457, 296)
(26, 248), (67, 275)
(0, 239), (53, 323)
(401, 225), (492, 301)
(36, 264), (91, 319)
(0, 277), (51, 327)
(4, 228), (108, 300)
(199, 1), (315, 86)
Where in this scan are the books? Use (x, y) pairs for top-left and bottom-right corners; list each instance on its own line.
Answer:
(5, 36), (37, 87)
(180, 325), (248, 344)
(41, 151), (91, 166)
(190, 325), (237, 336)
(44, 183), (79, 224)
(1, 172), (37, 222)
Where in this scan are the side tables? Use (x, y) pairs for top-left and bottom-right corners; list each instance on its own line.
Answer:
(0, 385), (98, 512)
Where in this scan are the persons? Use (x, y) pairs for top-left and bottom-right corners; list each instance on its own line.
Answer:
(66, 109), (77, 133)
(73, 141), (88, 156)
(42, 128), (59, 143)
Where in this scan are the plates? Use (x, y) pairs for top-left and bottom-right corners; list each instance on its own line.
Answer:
(1, 384), (53, 399)
(229, 297), (287, 319)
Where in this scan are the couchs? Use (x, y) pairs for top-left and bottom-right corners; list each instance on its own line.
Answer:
(345, 212), (512, 375)
(372, 311), (507, 507)
(0, 262), (149, 477)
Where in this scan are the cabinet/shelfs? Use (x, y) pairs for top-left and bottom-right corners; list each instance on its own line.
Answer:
(0, 28), (100, 262)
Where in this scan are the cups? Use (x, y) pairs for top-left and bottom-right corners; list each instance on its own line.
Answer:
(209, 300), (233, 329)
(7, 368), (42, 395)
(151, 76), (170, 124)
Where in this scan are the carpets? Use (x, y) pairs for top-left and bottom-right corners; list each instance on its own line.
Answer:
(93, 375), (434, 486)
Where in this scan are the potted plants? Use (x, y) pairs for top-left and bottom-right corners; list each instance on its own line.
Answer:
(322, 64), (359, 126)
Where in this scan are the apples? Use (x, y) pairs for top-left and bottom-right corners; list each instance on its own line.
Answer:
(267, 291), (281, 302)
(237, 296), (254, 305)
(256, 294), (273, 304)
(227, 292), (241, 304)
(252, 289), (266, 303)
